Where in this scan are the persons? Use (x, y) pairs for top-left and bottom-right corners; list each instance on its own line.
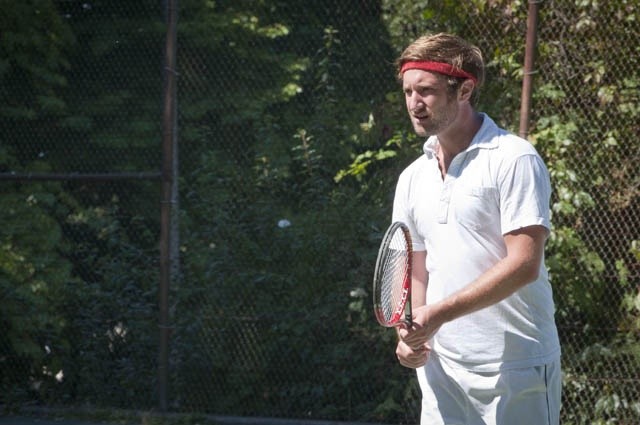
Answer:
(388, 33), (561, 425)
